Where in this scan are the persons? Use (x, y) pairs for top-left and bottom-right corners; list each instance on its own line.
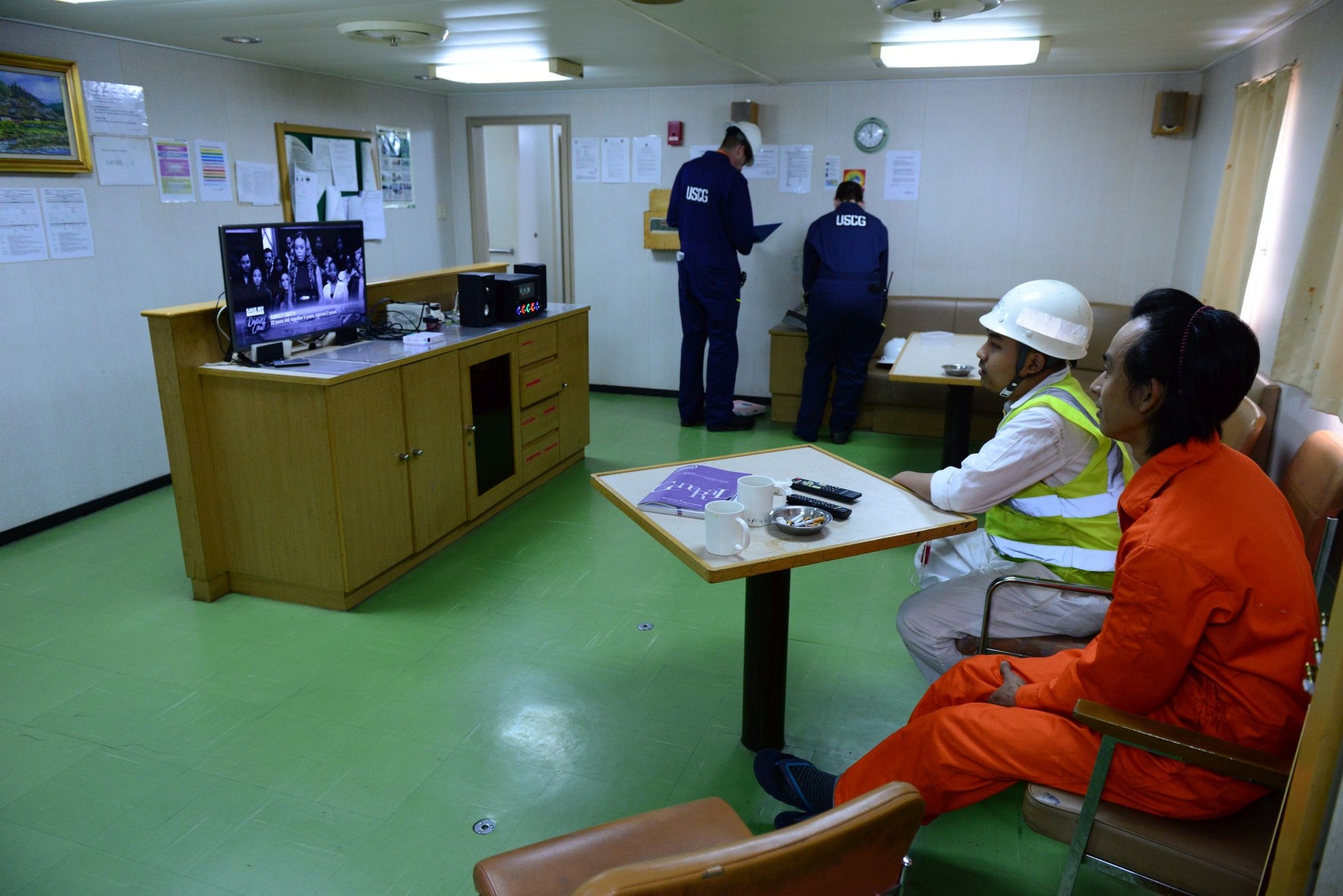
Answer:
(267, 255), (283, 297)
(355, 252), (361, 272)
(273, 270), (288, 311)
(337, 248), (356, 282)
(350, 256), (364, 302)
(666, 121), (762, 432)
(323, 260), (349, 306)
(229, 249), (253, 312)
(792, 180), (889, 445)
(288, 231), (323, 311)
(259, 247), (274, 281)
(891, 279), (1135, 690)
(241, 265), (272, 312)
(333, 235), (349, 272)
(281, 234), (293, 273)
(321, 257), (333, 287)
(754, 288), (1320, 818)
(312, 232), (329, 276)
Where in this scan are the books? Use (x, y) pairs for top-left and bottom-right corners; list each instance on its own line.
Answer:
(638, 465), (753, 520)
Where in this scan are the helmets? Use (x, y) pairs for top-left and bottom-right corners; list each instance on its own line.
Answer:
(726, 121), (762, 167)
(979, 280), (1093, 360)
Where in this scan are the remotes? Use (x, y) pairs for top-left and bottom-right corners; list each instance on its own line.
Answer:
(789, 477), (862, 501)
(786, 494), (852, 520)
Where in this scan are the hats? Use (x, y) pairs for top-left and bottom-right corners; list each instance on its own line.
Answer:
(836, 181), (863, 201)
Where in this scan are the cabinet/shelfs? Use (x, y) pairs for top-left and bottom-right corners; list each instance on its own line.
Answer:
(203, 307), (589, 611)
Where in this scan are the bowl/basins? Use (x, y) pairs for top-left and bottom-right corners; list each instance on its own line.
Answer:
(769, 505), (833, 536)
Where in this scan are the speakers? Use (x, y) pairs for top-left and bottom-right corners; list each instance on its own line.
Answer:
(1152, 91), (1189, 134)
(513, 263), (547, 310)
(458, 272), (496, 327)
(730, 102), (758, 126)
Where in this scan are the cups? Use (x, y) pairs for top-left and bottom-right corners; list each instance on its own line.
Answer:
(704, 501), (750, 555)
(737, 475), (786, 527)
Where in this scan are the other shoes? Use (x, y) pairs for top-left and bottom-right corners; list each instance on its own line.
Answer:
(831, 431), (850, 444)
(754, 752), (823, 813)
(774, 811), (815, 830)
(680, 416), (707, 427)
(792, 426), (818, 442)
(707, 413), (755, 431)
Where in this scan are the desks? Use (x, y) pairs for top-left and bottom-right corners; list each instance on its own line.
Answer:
(888, 331), (989, 469)
(590, 442), (977, 757)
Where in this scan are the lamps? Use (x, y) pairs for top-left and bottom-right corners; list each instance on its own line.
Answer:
(874, 0), (1004, 23)
(868, 36), (1053, 70)
(337, 21), (449, 48)
(429, 57), (583, 83)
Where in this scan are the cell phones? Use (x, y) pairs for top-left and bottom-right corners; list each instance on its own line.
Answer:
(273, 359), (309, 368)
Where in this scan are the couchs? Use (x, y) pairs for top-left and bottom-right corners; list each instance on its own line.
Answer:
(770, 296), (1279, 474)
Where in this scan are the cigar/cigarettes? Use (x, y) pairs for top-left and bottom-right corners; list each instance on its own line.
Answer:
(787, 513), (825, 527)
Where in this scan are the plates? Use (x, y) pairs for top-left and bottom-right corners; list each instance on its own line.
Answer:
(941, 364), (975, 376)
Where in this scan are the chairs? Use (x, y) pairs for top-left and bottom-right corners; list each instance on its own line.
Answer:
(1023, 562), (1343, 895)
(1221, 396), (1268, 456)
(955, 428), (1343, 655)
(472, 780), (922, 896)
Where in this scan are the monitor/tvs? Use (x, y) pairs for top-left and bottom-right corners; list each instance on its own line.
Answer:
(219, 221), (368, 350)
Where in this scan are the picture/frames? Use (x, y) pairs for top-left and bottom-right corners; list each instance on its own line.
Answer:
(0, 51), (95, 174)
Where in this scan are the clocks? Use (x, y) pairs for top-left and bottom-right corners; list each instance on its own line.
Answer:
(854, 117), (889, 153)
